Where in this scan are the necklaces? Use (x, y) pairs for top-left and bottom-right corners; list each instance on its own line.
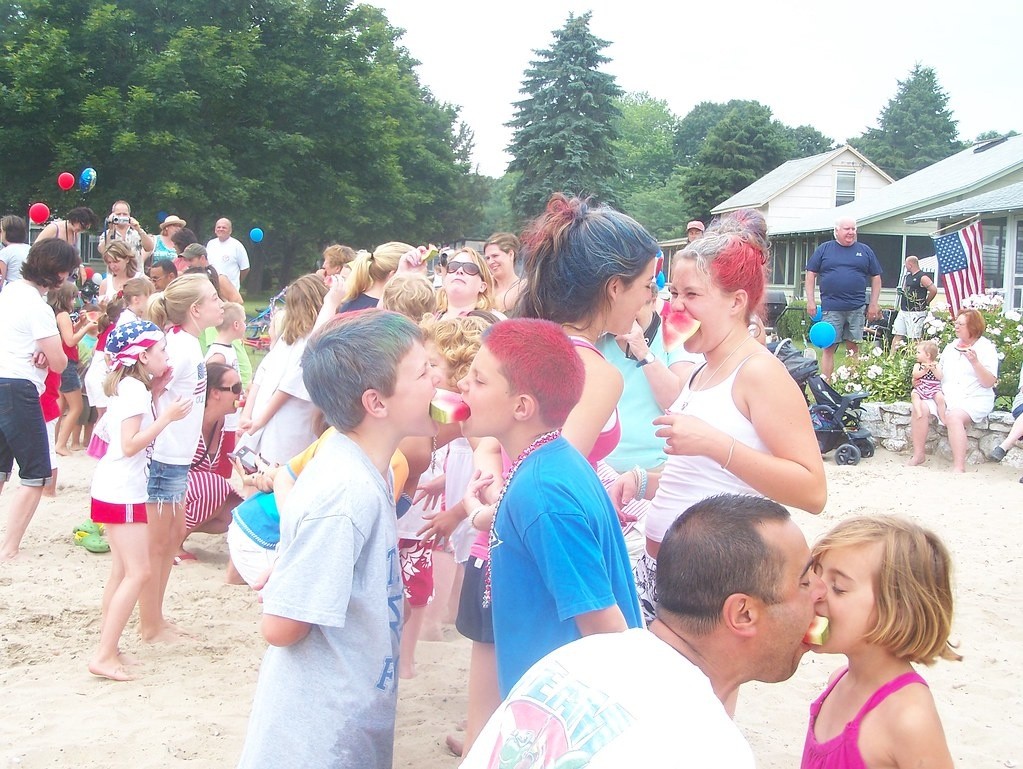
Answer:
(480, 429), (562, 610)
(681, 335), (752, 411)
(431, 435), (436, 474)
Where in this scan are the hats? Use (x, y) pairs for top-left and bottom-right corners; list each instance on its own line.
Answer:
(178, 244), (207, 259)
(686, 220), (705, 232)
(160, 215), (186, 230)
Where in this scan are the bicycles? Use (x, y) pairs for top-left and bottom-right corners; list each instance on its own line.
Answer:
(245, 308), (268, 337)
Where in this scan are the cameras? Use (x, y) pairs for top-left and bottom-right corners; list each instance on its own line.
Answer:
(112, 216), (129, 224)
(438, 249), (455, 267)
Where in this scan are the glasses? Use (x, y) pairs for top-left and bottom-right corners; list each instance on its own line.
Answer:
(209, 382), (243, 394)
(447, 261), (485, 282)
(151, 274), (170, 283)
(80, 221), (86, 230)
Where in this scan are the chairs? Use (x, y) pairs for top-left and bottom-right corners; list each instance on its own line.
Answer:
(858, 310), (892, 348)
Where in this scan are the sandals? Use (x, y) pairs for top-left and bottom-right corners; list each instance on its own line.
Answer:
(74, 532), (110, 552)
(992, 446), (1008, 461)
(73, 521), (104, 535)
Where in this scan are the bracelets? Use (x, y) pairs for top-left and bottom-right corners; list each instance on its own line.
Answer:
(467, 508), (481, 530)
(720, 435), (734, 470)
(631, 464), (646, 503)
(137, 229), (144, 235)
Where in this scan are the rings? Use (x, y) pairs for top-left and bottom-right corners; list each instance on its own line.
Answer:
(873, 316), (875, 318)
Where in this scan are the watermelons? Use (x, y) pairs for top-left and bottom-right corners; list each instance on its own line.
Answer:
(234, 399), (246, 408)
(803, 615), (831, 645)
(430, 388), (470, 424)
(85, 311), (99, 323)
(662, 312), (701, 354)
(955, 344), (971, 352)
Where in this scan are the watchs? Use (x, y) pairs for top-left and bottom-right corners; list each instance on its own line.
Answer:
(636, 352), (655, 369)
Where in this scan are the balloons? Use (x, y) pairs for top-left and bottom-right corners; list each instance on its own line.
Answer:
(157, 212), (168, 223)
(810, 322), (836, 349)
(79, 168), (96, 194)
(809, 305), (822, 322)
(250, 228), (263, 242)
(28, 203), (49, 224)
(57, 173), (74, 190)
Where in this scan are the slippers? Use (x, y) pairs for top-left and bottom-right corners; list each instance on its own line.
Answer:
(174, 549), (197, 565)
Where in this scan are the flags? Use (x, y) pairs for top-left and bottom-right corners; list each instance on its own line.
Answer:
(931, 220), (984, 322)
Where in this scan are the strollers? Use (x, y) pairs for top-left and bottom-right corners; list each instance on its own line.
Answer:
(765, 337), (875, 466)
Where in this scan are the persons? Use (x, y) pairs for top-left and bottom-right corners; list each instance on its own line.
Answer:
(458, 494), (828, 769)
(457, 319), (642, 700)
(593, 283), (695, 562)
(240, 309), (440, 769)
(992, 365), (1023, 483)
(911, 340), (947, 425)
(686, 221), (705, 245)
(886, 256), (937, 357)
(447, 191), (658, 761)
(799, 514), (962, 769)
(0, 199), (529, 680)
(908, 309), (997, 474)
(606, 208), (826, 722)
(805, 217), (883, 387)
